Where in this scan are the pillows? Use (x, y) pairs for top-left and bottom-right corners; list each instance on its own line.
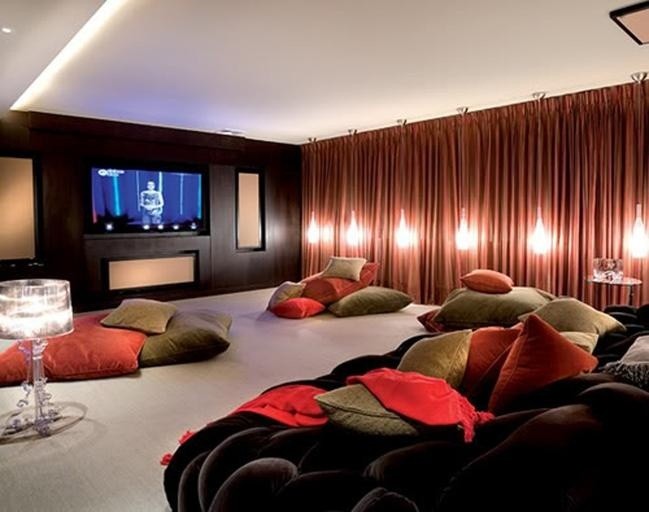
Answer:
(417, 306), (441, 334)
(98, 297), (181, 335)
(486, 312), (600, 411)
(321, 255), (369, 282)
(517, 294), (628, 337)
(299, 260), (382, 306)
(457, 268), (516, 296)
(462, 321), (525, 396)
(559, 330), (600, 354)
(265, 280), (308, 312)
(0, 309), (149, 390)
(328, 286), (415, 320)
(434, 287), (556, 329)
(272, 298), (325, 320)
(396, 328), (473, 398)
(138, 309), (235, 370)
(311, 383), (455, 438)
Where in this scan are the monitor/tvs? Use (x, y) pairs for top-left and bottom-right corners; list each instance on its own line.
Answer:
(87, 164), (207, 235)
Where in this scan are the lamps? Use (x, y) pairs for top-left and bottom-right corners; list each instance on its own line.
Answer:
(609, 0), (649, 45)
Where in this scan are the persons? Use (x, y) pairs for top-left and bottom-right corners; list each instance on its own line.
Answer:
(598, 259), (619, 274)
(139, 179), (164, 225)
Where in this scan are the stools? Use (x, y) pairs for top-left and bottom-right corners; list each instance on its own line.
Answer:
(1, 276), (91, 446)
